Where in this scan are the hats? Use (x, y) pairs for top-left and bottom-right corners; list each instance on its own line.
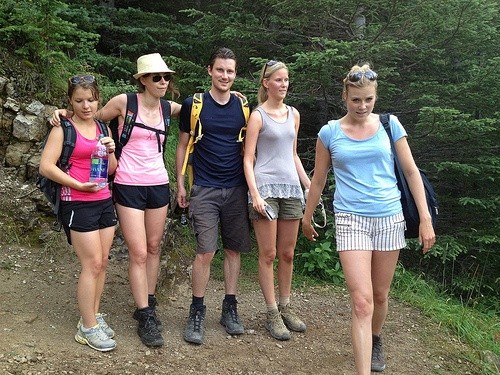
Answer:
(132, 52), (176, 80)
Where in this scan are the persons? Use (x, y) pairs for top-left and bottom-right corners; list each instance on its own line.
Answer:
(244, 59), (322, 341)
(39, 73), (119, 351)
(175, 46), (252, 345)
(49, 53), (249, 348)
(302, 64), (436, 375)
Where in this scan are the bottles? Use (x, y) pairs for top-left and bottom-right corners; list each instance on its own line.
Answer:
(89, 134), (108, 189)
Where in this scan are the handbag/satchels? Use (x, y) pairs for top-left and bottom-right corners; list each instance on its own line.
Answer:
(395, 169), (439, 238)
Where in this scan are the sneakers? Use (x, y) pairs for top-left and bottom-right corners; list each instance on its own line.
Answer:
(278, 303), (307, 332)
(183, 303), (206, 344)
(265, 307), (291, 341)
(74, 312), (118, 351)
(133, 294), (164, 346)
(219, 299), (244, 334)
(370, 333), (385, 371)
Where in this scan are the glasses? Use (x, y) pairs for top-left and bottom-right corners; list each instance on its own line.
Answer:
(150, 74), (171, 83)
(68, 75), (96, 85)
(262, 61), (276, 79)
(345, 71), (378, 91)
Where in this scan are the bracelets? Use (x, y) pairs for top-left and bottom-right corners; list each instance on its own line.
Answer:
(109, 150), (114, 154)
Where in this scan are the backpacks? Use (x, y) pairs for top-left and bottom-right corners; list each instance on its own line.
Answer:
(35, 117), (109, 207)
